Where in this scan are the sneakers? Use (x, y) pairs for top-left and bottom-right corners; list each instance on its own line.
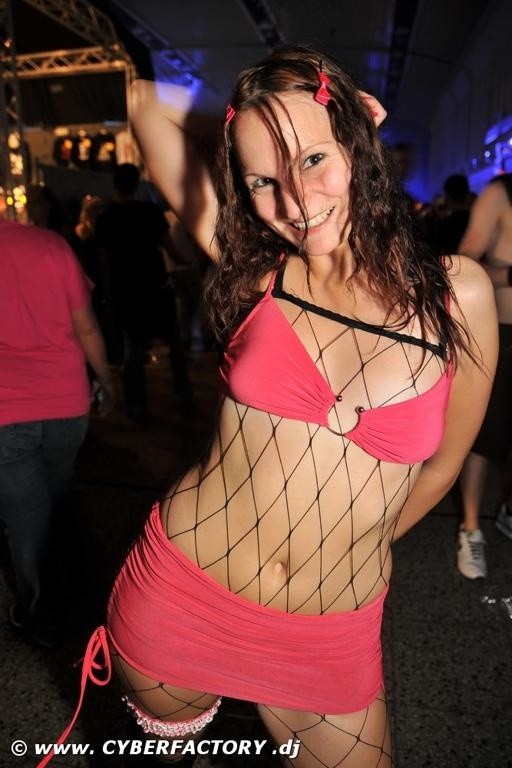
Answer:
(496, 503), (512, 537)
(456, 523), (488, 581)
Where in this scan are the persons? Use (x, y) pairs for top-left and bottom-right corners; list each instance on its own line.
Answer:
(101, 47), (502, 767)
(0, 128), (511, 640)
